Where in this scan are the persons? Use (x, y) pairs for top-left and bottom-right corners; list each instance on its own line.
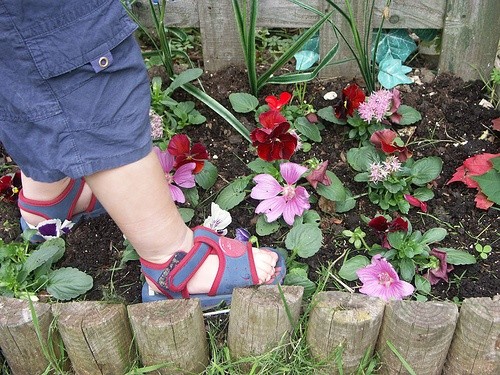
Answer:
(0, 0), (287, 311)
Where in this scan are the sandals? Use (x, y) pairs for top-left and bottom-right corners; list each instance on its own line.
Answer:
(140, 225), (287, 307)
(17, 176), (107, 243)
